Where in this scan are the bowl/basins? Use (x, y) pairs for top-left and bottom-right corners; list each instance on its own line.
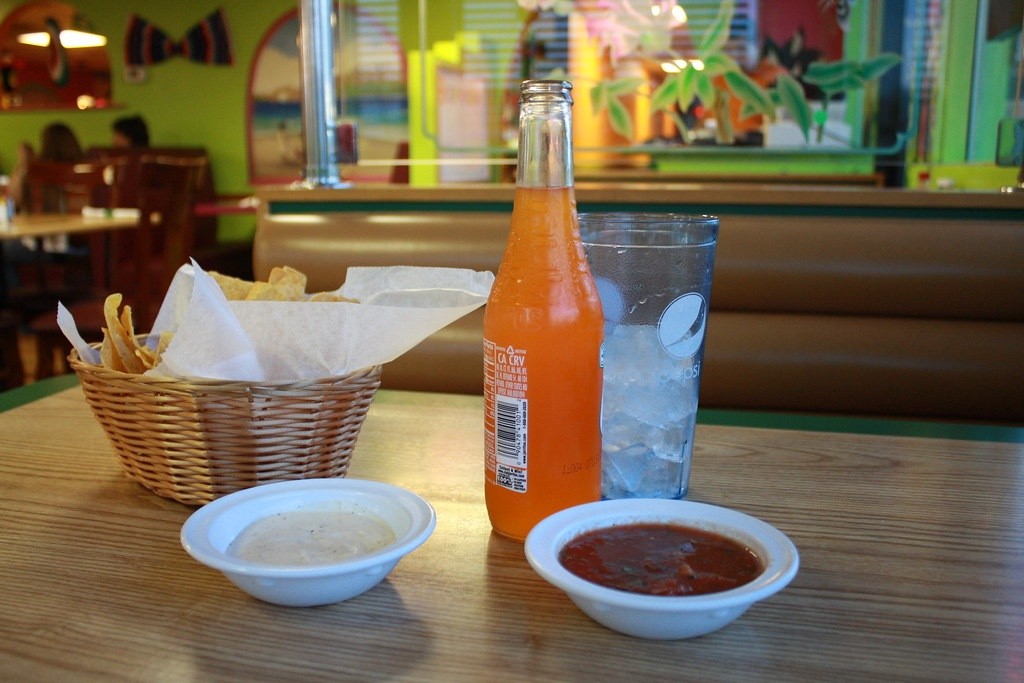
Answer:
(179, 477), (437, 607)
(524, 498), (799, 641)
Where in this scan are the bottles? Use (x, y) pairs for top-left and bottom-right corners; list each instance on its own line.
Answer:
(484, 78), (604, 540)
(0, 175), (16, 230)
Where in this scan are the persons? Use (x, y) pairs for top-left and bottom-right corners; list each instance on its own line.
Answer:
(0, 121), (85, 391)
(112, 115), (147, 147)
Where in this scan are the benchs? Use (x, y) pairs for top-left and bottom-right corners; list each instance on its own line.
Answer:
(253, 213), (1024, 445)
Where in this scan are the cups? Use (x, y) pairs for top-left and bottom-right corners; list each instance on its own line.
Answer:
(579, 211), (719, 501)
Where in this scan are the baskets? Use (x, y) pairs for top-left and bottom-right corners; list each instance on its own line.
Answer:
(67, 332), (382, 508)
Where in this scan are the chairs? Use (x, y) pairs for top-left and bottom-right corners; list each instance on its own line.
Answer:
(17, 143), (211, 384)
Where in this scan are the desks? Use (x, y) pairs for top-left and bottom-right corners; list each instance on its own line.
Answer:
(0, 383), (1023, 682)
(0, 211), (140, 235)
